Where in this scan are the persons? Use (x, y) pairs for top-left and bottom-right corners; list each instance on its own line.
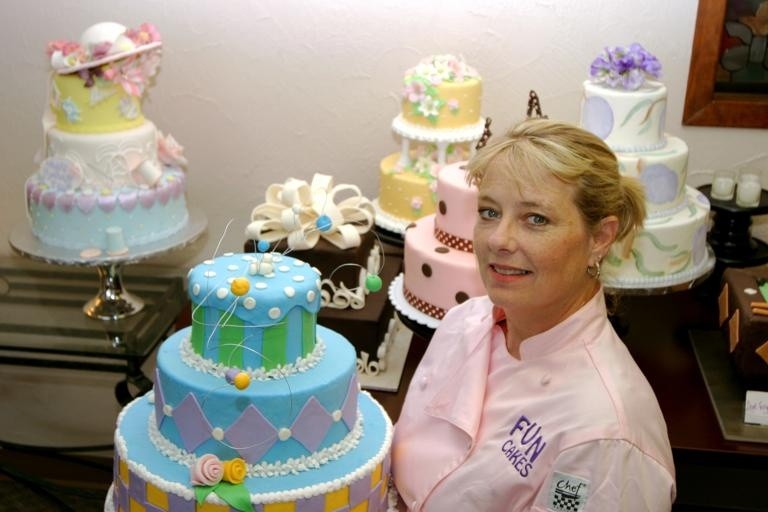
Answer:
(393, 119), (675, 512)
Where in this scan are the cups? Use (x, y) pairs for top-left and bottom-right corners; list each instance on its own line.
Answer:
(711, 170), (736, 200)
(737, 169), (762, 206)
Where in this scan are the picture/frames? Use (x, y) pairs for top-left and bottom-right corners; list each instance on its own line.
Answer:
(681, 0), (768, 129)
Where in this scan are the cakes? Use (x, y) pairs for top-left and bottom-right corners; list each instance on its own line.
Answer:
(401, 115), (488, 320)
(21, 21), (192, 258)
(577, 41), (716, 286)
(378, 51), (491, 222)
(103, 203), (397, 512)
(242, 172), (382, 310)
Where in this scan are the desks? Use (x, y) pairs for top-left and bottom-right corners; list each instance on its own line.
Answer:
(0, 348), (157, 512)
(696, 184), (767, 267)
